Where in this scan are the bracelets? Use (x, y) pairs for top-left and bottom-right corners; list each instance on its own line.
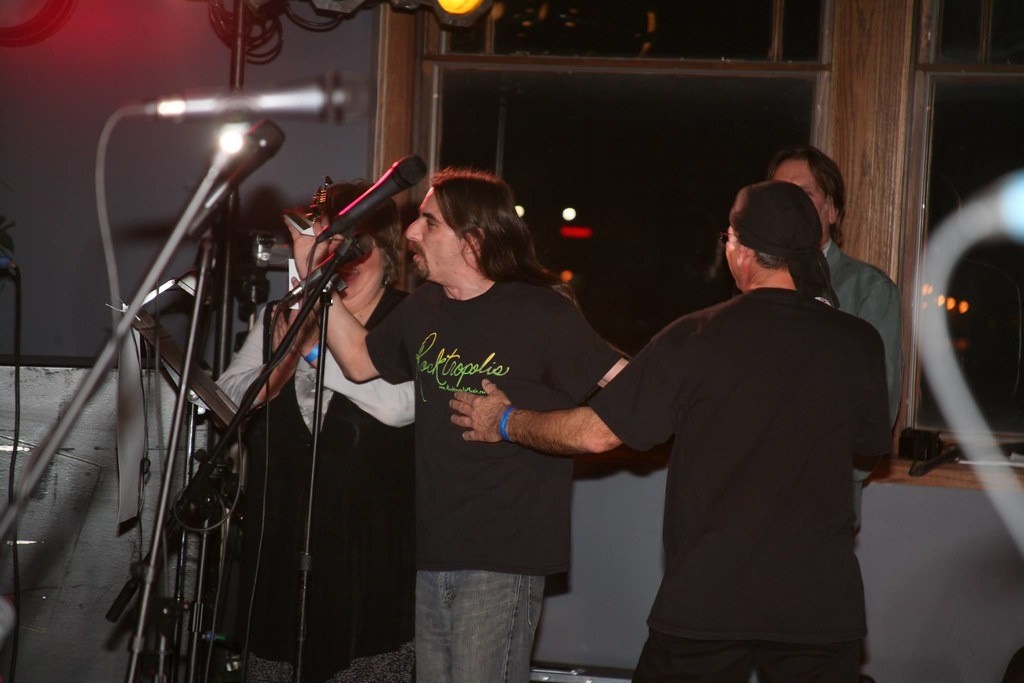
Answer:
(500, 407), (512, 441)
(305, 343), (319, 364)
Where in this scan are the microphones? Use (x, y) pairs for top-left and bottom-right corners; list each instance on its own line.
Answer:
(183, 121), (286, 241)
(145, 69), (372, 127)
(279, 232), (374, 307)
(316, 156), (428, 242)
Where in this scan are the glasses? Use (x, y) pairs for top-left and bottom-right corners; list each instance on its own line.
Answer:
(718, 231), (728, 244)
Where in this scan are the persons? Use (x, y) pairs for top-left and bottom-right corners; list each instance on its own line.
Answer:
(284, 169), (632, 682)
(767, 144), (904, 538)
(449, 181), (890, 683)
(211, 178), (415, 683)
